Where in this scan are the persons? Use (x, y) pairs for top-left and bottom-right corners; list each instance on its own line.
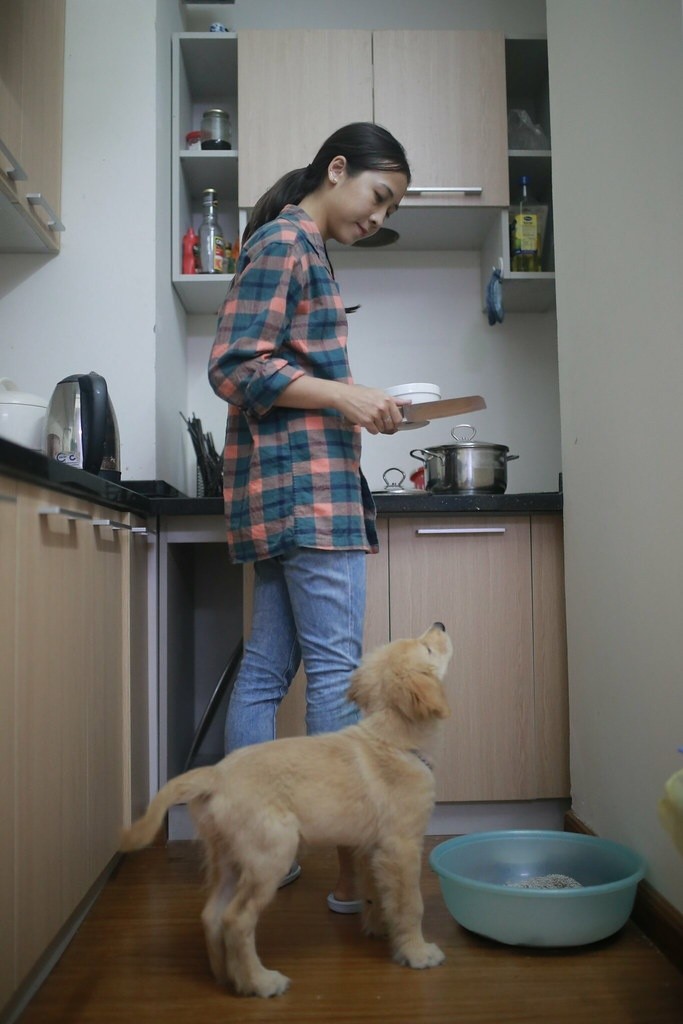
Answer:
(208, 123), (411, 913)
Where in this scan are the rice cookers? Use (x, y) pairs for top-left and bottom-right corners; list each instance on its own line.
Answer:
(0, 377), (47, 452)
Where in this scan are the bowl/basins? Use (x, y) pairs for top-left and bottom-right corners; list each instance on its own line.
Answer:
(385, 383), (440, 405)
(431, 830), (645, 945)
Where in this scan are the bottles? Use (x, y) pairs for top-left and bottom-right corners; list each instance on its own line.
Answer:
(183, 188), (236, 274)
(512, 176), (541, 271)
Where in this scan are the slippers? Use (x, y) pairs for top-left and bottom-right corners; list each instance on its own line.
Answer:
(327, 894), (366, 913)
(277, 861), (302, 889)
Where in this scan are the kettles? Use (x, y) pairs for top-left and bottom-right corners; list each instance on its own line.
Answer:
(44, 371), (121, 484)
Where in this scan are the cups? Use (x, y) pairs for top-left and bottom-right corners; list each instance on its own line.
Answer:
(211, 22), (225, 33)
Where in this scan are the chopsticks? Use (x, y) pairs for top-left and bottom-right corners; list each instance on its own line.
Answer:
(180, 412), (225, 496)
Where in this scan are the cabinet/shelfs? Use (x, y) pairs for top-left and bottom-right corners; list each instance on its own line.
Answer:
(0, 476), (571, 1008)
(171, 30), (557, 318)
(0, 0), (69, 254)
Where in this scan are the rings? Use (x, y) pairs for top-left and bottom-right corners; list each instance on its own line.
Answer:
(382, 416), (392, 424)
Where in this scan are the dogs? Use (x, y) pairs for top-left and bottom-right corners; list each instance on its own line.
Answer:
(116, 619), (454, 998)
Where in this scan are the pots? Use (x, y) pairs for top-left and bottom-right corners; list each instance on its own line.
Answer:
(411, 424), (518, 494)
